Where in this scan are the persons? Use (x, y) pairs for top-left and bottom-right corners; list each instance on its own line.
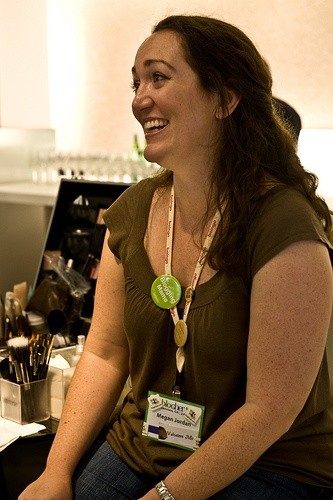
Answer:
(17, 15), (333, 500)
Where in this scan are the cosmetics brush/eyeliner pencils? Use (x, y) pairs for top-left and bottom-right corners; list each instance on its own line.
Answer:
(0, 250), (101, 425)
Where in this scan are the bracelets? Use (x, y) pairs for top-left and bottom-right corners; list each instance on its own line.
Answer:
(155, 480), (177, 500)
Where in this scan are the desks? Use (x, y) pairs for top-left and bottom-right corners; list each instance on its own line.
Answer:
(0, 416), (61, 454)
(0, 179), (60, 307)
